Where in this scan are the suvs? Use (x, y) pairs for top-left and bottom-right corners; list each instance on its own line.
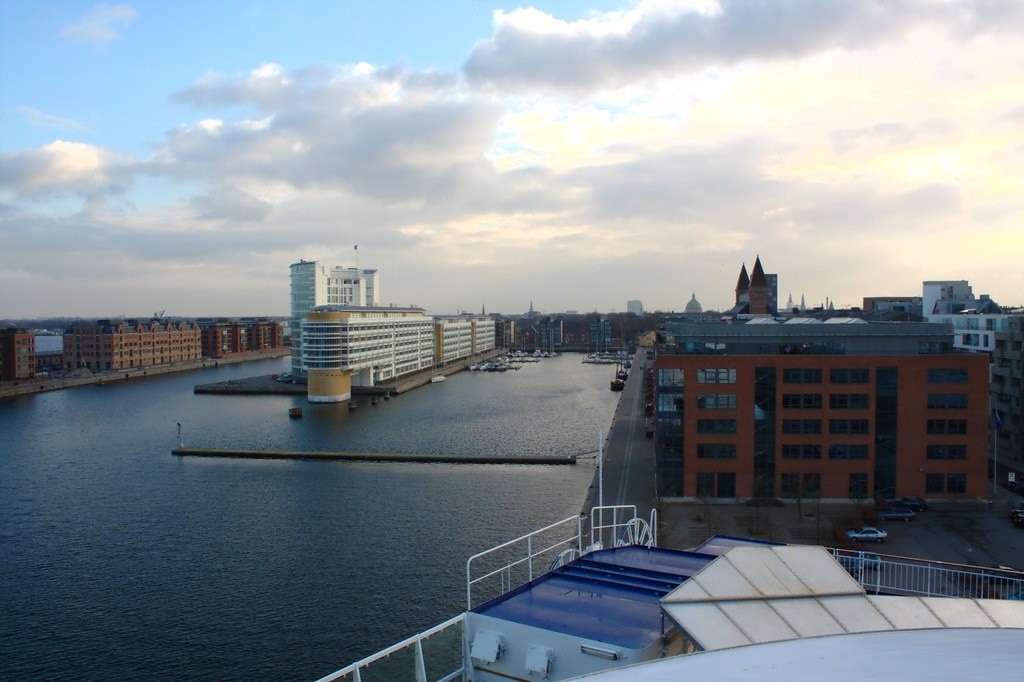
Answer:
(892, 496), (929, 512)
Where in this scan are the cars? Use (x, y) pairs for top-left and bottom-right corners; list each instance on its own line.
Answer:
(1008, 507), (1024, 527)
(878, 506), (918, 522)
(845, 525), (888, 543)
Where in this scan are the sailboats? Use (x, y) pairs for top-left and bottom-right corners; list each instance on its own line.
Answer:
(430, 351), (446, 383)
(468, 327), (632, 391)
